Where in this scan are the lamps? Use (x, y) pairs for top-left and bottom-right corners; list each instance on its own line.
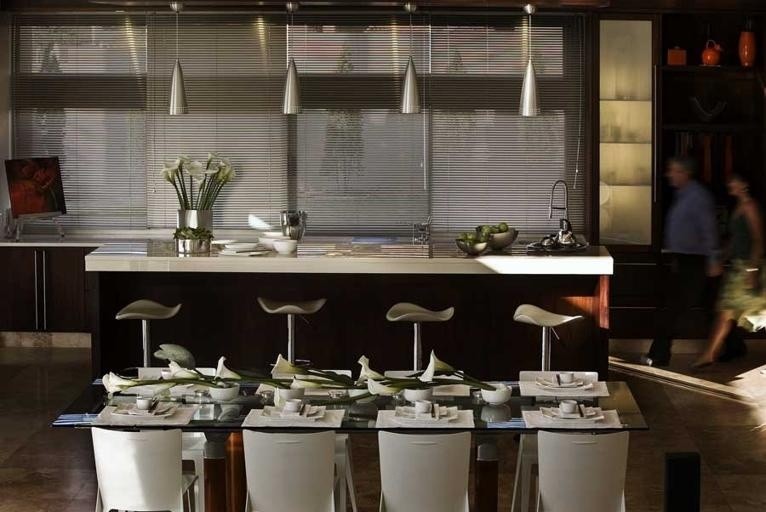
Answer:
(400, 1), (421, 115)
(282, 1), (302, 116)
(168, 1), (188, 116)
(518, 1), (539, 118)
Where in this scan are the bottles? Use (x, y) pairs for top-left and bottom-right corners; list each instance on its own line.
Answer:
(738, 31), (755, 67)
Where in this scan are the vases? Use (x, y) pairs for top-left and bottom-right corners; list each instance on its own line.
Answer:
(176, 210), (214, 235)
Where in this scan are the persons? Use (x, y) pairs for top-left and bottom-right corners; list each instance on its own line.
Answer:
(642, 151), (751, 367)
(688, 156), (766, 372)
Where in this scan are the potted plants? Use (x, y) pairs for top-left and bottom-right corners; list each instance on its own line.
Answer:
(171, 227), (215, 254)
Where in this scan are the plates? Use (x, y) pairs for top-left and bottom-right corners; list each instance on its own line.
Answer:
(111, 396), (176, 418)
(392, 383), (459, 421)
(226, 241), (258, 250)
(260, 369), (326, 422)
(536, 372), (604, 423)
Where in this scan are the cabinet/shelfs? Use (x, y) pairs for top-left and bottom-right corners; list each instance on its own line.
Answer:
(0, 246), (98, 333)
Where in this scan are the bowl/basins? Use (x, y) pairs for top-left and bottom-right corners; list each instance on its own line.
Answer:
(261, 231), (297, 254)
(455, 225), (519, 254)
(209, 382), (240, 402)
(174, 237), (210, 253)
(481, 385), (513, 406)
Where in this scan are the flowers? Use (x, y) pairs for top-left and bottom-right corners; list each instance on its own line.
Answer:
(159, 151), (237, 209)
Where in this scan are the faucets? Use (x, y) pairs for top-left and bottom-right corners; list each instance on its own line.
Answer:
(548, 180), (572, 230)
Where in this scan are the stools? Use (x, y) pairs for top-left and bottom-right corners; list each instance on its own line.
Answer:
(112, 298), (184, 363)
(383, 300), (456, 371)
(511, 301), (585, 371)
(257, 297), (329, 361)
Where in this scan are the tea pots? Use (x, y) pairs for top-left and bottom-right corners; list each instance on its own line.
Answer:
(703, 38), (725, 65)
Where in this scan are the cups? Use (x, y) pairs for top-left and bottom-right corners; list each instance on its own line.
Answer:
(281, 210), (307, 242)
(161, 368), (174, 380)
